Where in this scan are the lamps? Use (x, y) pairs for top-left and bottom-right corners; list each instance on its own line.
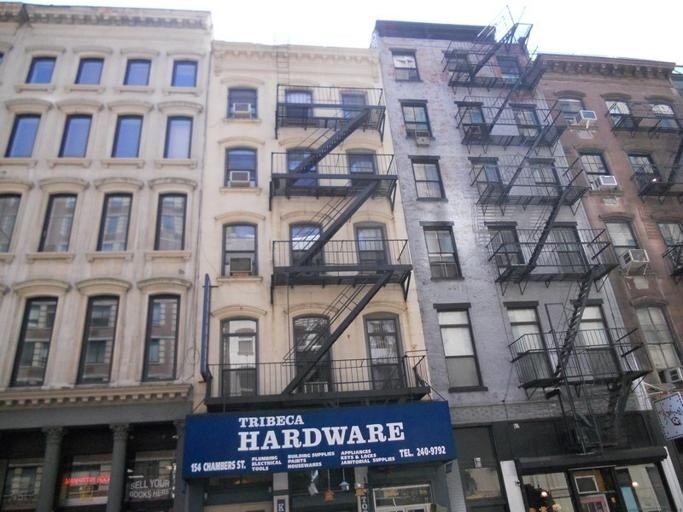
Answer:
(323, 469), (335, 503)
(511, 423), (519, 430)
(338, 470), (351, 490)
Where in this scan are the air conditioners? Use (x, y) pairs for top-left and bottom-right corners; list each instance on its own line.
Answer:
(592, 175), (617, 191)
(228, 255), (255, 275)
(616, 248), (652, 278)
(576, 110), (597, 125)
(664, 367), (683, 383)
(229, 169), (253, 188)
(232, 101), (252, 116)
